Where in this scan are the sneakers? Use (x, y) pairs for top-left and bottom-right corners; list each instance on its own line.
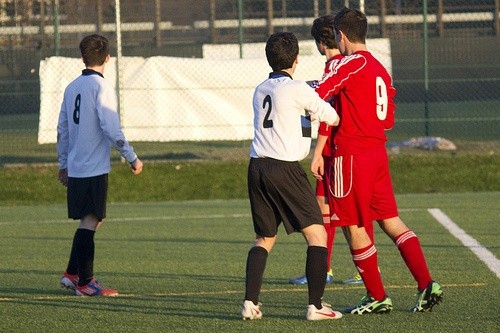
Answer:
(343, 272), (364, 285)
(345, 294), (392, 314)
(413, 281), (444, 312)
(75, 279), (118, 297)
(242, 300), (262, 320)
(60, 271), (79, 291)
(306, 302), (342, 320)
(289, 269), (332, 285)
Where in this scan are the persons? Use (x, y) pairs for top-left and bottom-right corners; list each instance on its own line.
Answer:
(241, 31), (343, 320)
(57, 33), (143, 296)
(289, 14), (363, 284)
(311, 6), (444, 314)
